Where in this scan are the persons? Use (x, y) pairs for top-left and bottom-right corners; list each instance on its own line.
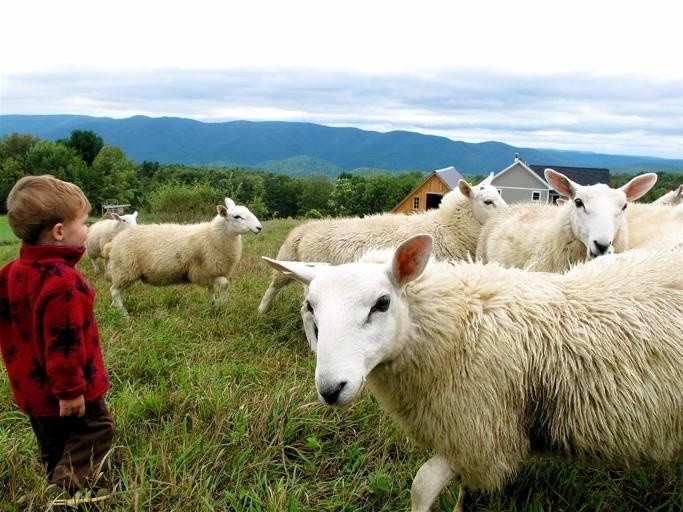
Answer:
(0, 176), (113, 481)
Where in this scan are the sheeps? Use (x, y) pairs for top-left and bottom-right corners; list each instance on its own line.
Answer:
(256, 167), (682, 512)
(84, 210), (139, 275)
(101, 196), (264, 320)
